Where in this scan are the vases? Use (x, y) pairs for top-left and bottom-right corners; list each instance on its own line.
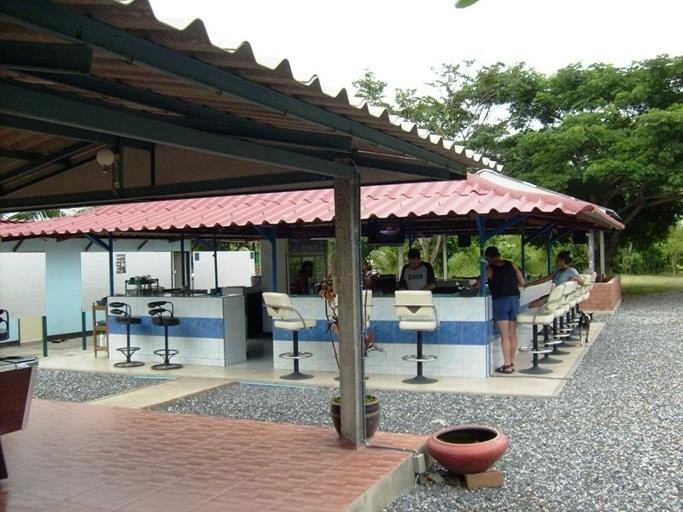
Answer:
(424, 424), (510, 476)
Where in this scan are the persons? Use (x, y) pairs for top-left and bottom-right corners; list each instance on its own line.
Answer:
(398, 247), (437, 290)
(292, 260), (315, 295)
(526, 250), (586, 307)
(472, 246), (525, 374)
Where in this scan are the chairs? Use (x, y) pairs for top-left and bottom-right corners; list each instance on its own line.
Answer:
(515, 270), (597, 377)
(108, 301), (144, 369)
(261, 289), (318, 382)
(146, 299), (181, 375)
(332, 287), (372, 385)
(391, 289), (442, 387)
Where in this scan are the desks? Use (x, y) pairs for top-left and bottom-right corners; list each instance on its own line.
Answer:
(0, 360), (38, 485)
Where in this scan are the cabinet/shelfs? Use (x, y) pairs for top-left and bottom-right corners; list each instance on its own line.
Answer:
(91, 301), (108, 359)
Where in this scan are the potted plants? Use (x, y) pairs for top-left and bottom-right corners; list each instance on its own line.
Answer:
(319, 259), (385, 441)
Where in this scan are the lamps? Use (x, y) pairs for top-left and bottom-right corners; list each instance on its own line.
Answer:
(92, 146), (119, 176)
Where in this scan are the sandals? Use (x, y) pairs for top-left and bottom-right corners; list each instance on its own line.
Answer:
(495, 363), (515, 374)
